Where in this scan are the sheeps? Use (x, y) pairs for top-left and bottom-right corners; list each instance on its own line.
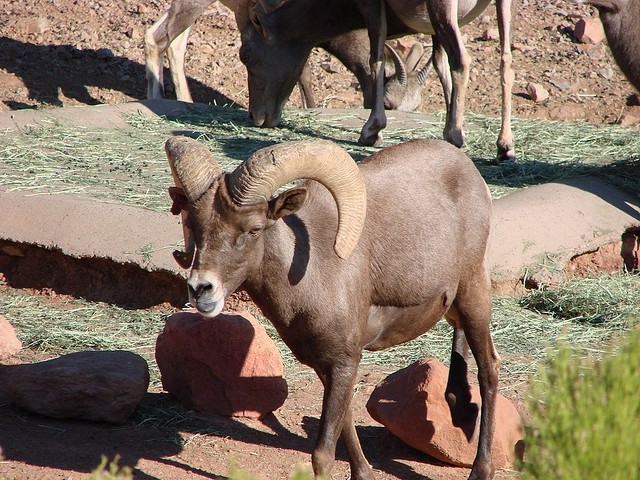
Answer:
(162, 131), (502, 480)
(140, 0), (640, 166)
